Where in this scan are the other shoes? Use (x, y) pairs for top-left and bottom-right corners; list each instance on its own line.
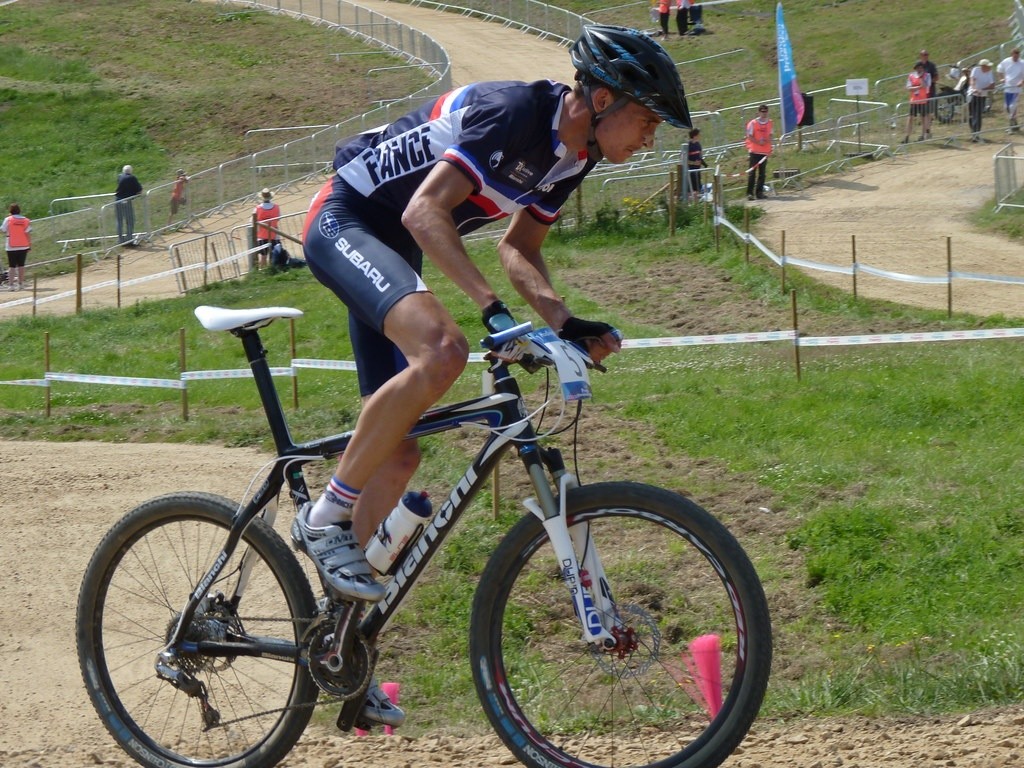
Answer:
(7, 285), (15, 291)
(748, 195), (754, 200)
(901, 138), (909, 144)
(924, 133), (932, 140)
(756, 194), (768, 199)
(1005, 127), (1018, 135)
(973, 136), (979, 143)
(17, 285), (24, 289)
(913, 136), (924, 143)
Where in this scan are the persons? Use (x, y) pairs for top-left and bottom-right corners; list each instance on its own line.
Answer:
(675, 0), (690, 36)
(966, 59), (995, 142)
(899, 61), (931, 144)
(249, 188), (280, 269)
(291, 23), (693, 726)
(166, 169), (190, 225)
(996, 48), (1024, 135)
(1, 203), (32, 292)
(115, 165), (142, 246)
(688, 129), (708, 202)
(657, 0), (671, 40)
(745, 106), (774, 201)
(920, 50), (939, 139)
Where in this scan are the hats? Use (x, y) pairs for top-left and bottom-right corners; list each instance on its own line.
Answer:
(979, 59), (994, 67)
(258, 188), (275, 201)
(176, 169), (187, 177)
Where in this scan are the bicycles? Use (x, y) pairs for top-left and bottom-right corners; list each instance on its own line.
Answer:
(72, 305), (775, 768)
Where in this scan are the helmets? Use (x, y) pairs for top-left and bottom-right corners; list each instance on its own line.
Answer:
(568, 24), (692, 128)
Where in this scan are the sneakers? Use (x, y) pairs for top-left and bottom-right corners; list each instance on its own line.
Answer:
(290, 501), (387, 600)
(362, 675), (405, 727)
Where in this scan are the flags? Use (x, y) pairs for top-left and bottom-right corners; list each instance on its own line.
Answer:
(776, 4), (804, 136)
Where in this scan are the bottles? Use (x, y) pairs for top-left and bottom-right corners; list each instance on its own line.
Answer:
(364, 489), (433, 576)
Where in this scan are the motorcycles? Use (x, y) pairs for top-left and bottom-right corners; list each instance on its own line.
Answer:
(934, 63), (995, 123)
